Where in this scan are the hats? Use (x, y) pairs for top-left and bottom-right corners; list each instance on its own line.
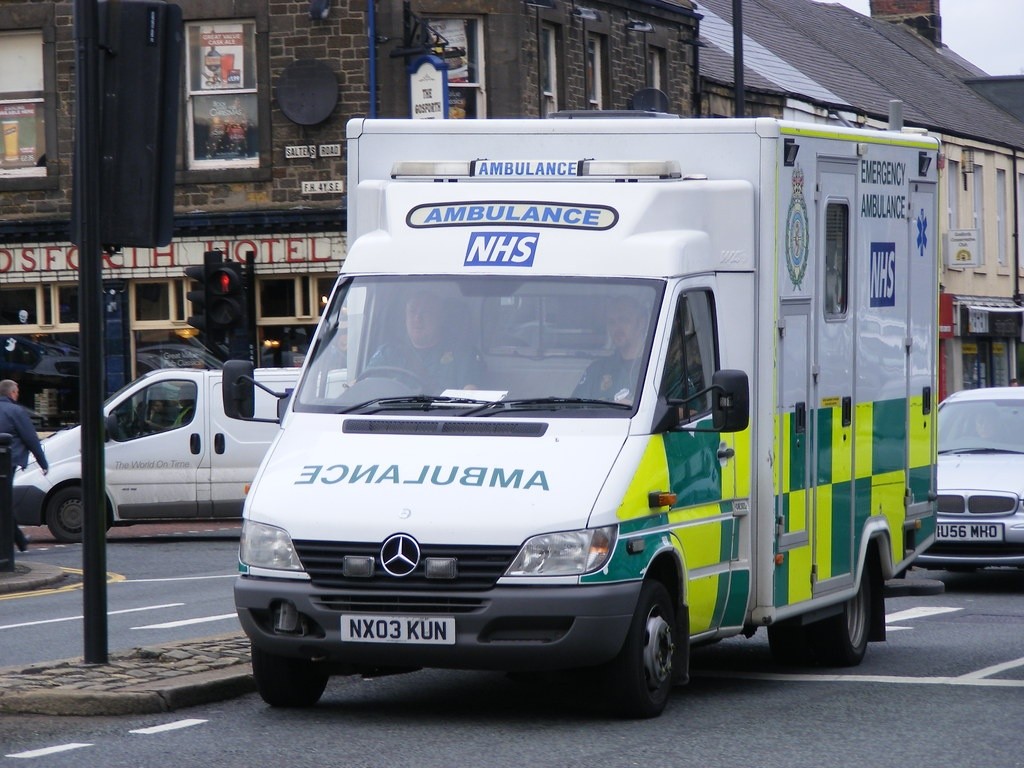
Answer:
(172, 383), (196, 394)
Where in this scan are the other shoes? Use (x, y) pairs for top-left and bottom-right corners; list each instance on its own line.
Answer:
(18, 534), (34, 553)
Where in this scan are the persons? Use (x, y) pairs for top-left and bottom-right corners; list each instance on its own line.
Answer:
(0, 379), (48, 552)
(349, 291), (486, 394)
(976, 410), (999, 438)
(567, 296), (701, 421)
(143, 383), (194, 430)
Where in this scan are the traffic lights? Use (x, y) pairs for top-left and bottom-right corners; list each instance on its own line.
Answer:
(205, 261), (252, 365)
(183, 250), (222, 350)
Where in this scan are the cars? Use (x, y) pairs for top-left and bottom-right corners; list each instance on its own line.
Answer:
(1, 330), (230, 469)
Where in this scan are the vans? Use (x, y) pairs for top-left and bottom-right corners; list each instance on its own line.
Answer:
(908, 386), (1024, 589)
(10, 357), (348, 544)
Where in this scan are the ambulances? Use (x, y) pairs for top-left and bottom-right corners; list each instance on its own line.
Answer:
(220, 108), (948, 718)
